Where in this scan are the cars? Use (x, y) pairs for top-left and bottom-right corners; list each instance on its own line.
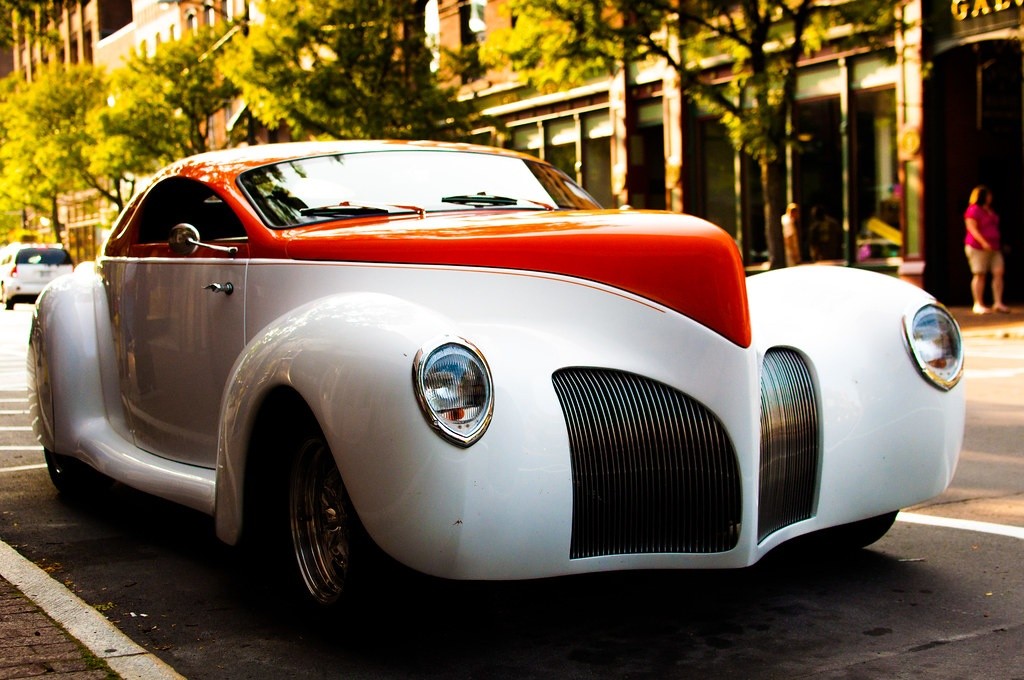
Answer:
(0, 242), (74, 310)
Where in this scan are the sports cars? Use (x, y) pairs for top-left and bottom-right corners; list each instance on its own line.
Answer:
(28, 136), (968, 657)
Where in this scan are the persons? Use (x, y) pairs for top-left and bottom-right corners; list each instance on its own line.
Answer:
(963, 187), (1010, 314)
(781, 204), (845, 265)
